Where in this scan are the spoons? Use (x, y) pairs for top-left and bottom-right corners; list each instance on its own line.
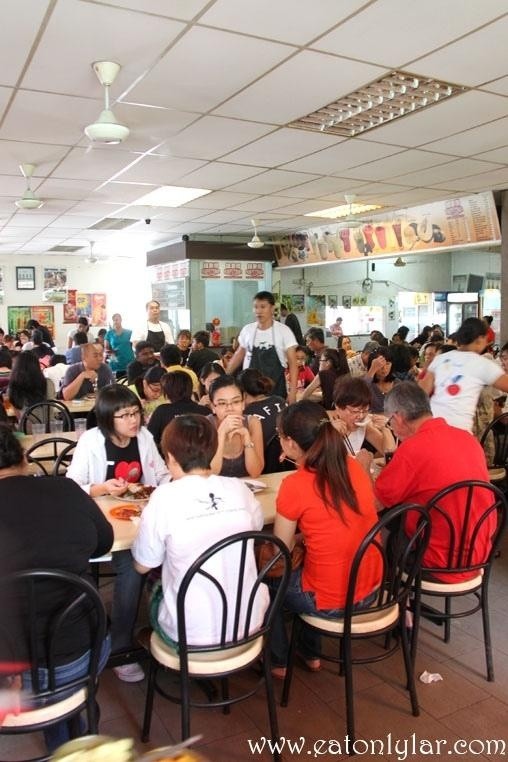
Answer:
(354, 419), (372, 425)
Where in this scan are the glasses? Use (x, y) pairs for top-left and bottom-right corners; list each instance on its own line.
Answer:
(346, 408), (373, 416)
(113, 409), (143, 419)
(211, 398), (244, 409)
(385, 410), (398, 428)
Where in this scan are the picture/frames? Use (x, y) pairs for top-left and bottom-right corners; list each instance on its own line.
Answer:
(16, 266), (35, 290)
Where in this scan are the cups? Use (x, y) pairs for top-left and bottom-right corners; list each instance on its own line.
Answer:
(384, 447), (397, 463)
(74, 418), (87, 438)
(32, 424), (46, 442)
(49, 420), (63, 438)
(308, 221), (404, 261)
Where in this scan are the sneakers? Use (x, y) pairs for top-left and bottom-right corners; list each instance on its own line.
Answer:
(113, 660), (145, 683)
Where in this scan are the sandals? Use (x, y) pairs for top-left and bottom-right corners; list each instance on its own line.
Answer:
(271, 666), (288, 680)
(302, 656), (325, 674)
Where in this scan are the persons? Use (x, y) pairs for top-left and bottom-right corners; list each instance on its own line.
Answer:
(258, 401), (387, 680)
(1, 427), (115, 756)
(64, 383), (176, 683)
(0, 291), (505, 472)
(134, 412), (265, 679)
(375, 381), (498, 643)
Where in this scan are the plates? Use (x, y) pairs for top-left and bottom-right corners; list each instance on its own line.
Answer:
(244, 479), (265, 493)
(109, 504), (142, 520)
(112, 483), (156, 501)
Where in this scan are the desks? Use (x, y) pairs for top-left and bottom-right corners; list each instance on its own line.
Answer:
(20, 431), (83, 476)
(80, 468), (507, 668)
(54, 397), (96, 417)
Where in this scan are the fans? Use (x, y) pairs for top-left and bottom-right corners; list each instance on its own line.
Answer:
(85, 240), (98, 264)
(339, 194), (361, 229)
(83, 60), (131, 146)
(14, 163), (44, 211)
(247, 218), (265, 249)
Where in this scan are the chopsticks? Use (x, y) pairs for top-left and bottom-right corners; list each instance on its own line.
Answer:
(332, 414), (355, 457)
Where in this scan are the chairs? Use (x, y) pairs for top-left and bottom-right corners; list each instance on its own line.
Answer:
(20, 400), (75, 432)
(53, 442), (77, 475)
(480, 411), (508, 465)
(0, 569), (111, 762)
(141, 530), (293, 761)
(396, 480), (508, 689)
(280, 503), (432, 757)
(25, 438), (75, 476)
